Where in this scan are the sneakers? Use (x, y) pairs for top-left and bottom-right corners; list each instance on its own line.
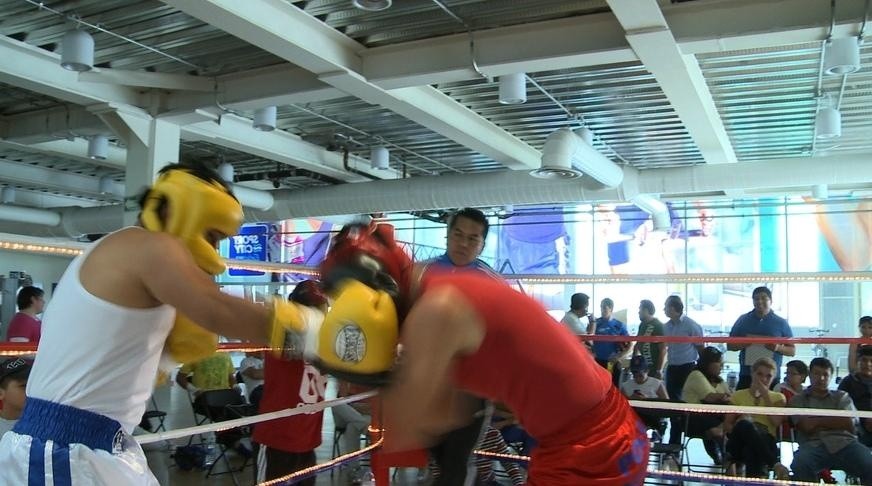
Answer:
(350, 465), (364, 486)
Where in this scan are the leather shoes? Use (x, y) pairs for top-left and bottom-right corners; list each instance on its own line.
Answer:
(233, 442), (253, 458)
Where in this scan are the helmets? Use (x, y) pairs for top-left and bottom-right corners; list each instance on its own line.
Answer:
(139, 164), (247, 278)
(318, 220), (414, 320)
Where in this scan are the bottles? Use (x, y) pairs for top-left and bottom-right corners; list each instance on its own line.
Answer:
(206, 431), (216, 466)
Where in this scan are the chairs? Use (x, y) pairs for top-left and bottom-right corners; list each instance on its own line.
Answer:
(331, 427), (372, 480)
(649, 421), (795, 478)
(142, 375), (263, 485)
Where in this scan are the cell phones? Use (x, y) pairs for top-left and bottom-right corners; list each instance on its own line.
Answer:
(633, 389), (641, 394)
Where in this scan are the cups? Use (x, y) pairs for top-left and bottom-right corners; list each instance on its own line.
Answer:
(727, 372), (739, 389)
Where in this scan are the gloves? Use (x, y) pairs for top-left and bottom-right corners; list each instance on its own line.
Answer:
(154, 306), (220, 390)
(266, 278), (401, 374)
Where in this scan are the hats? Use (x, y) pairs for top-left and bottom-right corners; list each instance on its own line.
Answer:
(630, 355), (648, 372)
(856, 343), (872, 356)
(0, 357), (32, 380)
